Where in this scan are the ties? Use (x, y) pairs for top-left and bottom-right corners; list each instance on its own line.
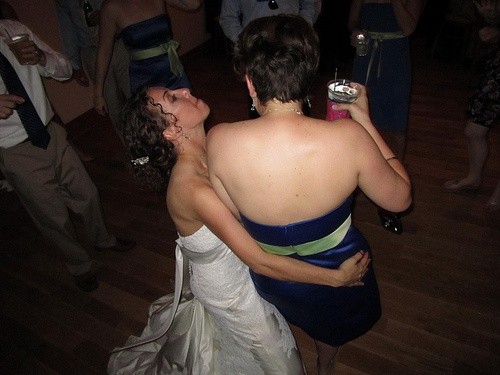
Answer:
(0, 51), (51, 150)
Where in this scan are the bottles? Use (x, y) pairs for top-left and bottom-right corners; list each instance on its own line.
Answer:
(83, 0), (97, 27)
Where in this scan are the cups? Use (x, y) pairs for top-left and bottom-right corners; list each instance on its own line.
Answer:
(353, 32), (368, 57)
(4, 34), (31, 65)
(326, 79), (360, 121)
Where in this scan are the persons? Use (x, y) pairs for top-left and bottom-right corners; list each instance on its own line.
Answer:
(0, 17), (137, 291)
(93, 0), (200, 116)
(120, 79), (372, 375)
(205, 12), (415, 375)
(441, 0), (500, 211)
(219, 0), (322, 121)
(56, 0), (139, 153)
(347, 0), (421, 234)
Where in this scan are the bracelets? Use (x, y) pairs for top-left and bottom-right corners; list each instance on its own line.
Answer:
(386, 156), (399, 161)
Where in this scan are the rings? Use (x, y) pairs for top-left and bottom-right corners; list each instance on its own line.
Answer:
(34, 50), (40, 57)
(359, 274), (363, 281)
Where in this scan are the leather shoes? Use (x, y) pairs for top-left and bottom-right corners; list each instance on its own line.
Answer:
(377, 209), (402, 234)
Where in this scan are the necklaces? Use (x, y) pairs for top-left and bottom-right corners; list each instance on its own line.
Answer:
(177, 152), (209, 159)
(263, 105), (304, 117)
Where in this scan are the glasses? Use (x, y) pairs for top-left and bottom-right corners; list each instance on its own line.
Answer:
(267, 0), (278, 9)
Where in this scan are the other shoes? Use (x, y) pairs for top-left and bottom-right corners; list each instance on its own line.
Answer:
(95, 237), (137, 252)
(74, 271), (99, 293)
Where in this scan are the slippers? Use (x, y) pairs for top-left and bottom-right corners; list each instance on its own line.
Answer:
(440, 177), (482, 194)
